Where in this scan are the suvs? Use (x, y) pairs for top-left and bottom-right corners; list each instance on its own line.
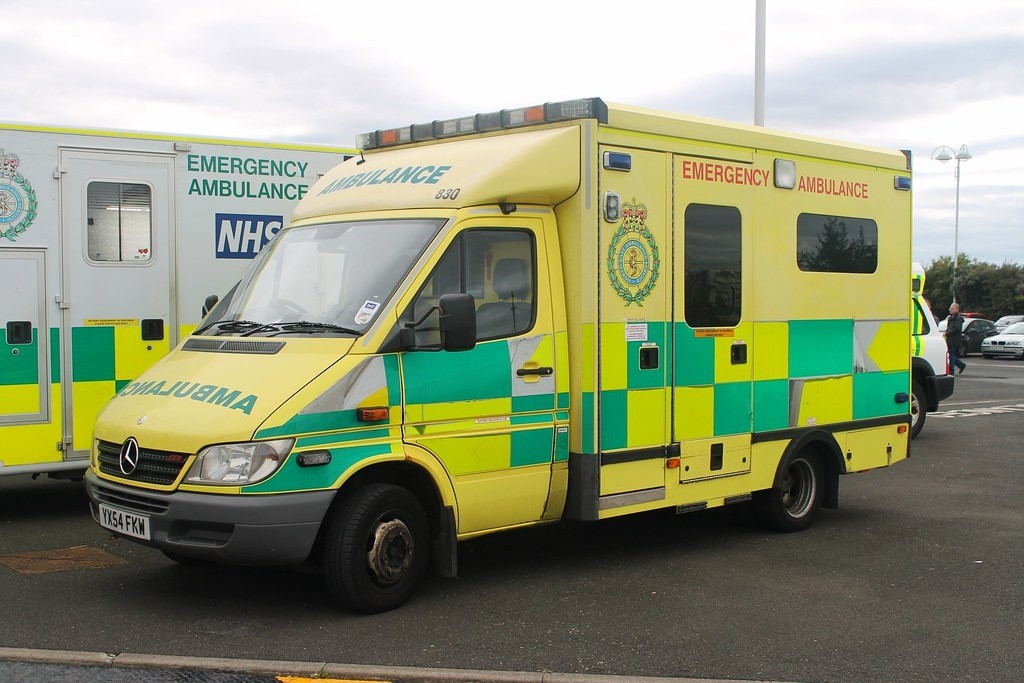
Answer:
(911, 263), (955, 443)
(946, 312), (994, 319)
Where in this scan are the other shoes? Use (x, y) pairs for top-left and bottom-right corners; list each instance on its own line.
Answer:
(949, 371), (954, 375)
(959, 363), (966, 374)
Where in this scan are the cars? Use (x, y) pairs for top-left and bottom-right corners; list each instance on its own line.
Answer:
(980, 322), (1024, 361)
(994, 315), (1024, 334)
(937, 318), (998, 357)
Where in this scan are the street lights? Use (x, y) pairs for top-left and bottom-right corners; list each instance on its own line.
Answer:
(930, 143), (972, 304)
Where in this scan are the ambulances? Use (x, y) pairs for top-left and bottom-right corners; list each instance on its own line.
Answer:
(82, 98), (914, 617)
(0, 121), (361, 488)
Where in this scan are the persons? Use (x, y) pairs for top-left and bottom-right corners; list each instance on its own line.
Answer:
(923, 299), (967, 377)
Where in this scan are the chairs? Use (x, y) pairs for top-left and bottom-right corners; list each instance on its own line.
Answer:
(476, 258), (533, 338)
